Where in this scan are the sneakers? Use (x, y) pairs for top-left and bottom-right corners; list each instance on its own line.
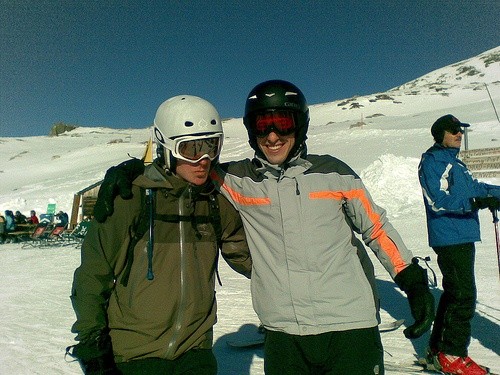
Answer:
(433, 352), (488, 375)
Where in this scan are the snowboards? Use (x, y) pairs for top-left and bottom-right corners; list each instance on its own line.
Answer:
(226, 319), (405, 348)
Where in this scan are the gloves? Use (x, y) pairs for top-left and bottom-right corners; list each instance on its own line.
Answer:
(73, 334), (122, 375)
(394, 262), (435, 339)
(478, 197), (500, 211)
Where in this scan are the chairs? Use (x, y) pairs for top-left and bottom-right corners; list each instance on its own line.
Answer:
(7, 218), (90, 250)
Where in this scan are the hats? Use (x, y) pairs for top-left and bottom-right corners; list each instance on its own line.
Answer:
(431, 114), (469, 136)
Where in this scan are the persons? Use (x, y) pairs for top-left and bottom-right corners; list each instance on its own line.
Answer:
(417, 114), (500, 375)
(49, 211), (69, 235)
(26, 210), (39, 224)
(92, 80), (435, 375)
(16, 210), (28, 224)
(70, 96), (253, 375)
(0, 209), (17, 244)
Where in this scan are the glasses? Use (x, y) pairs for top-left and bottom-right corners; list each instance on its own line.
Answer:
(447, 129), (464, 135)
(253, 113), (295, 136)
(155, 124), (224, 169)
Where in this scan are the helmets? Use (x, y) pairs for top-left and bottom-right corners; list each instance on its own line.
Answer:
(155, 95), (223, 148)
(243, 79), (309, 152)
